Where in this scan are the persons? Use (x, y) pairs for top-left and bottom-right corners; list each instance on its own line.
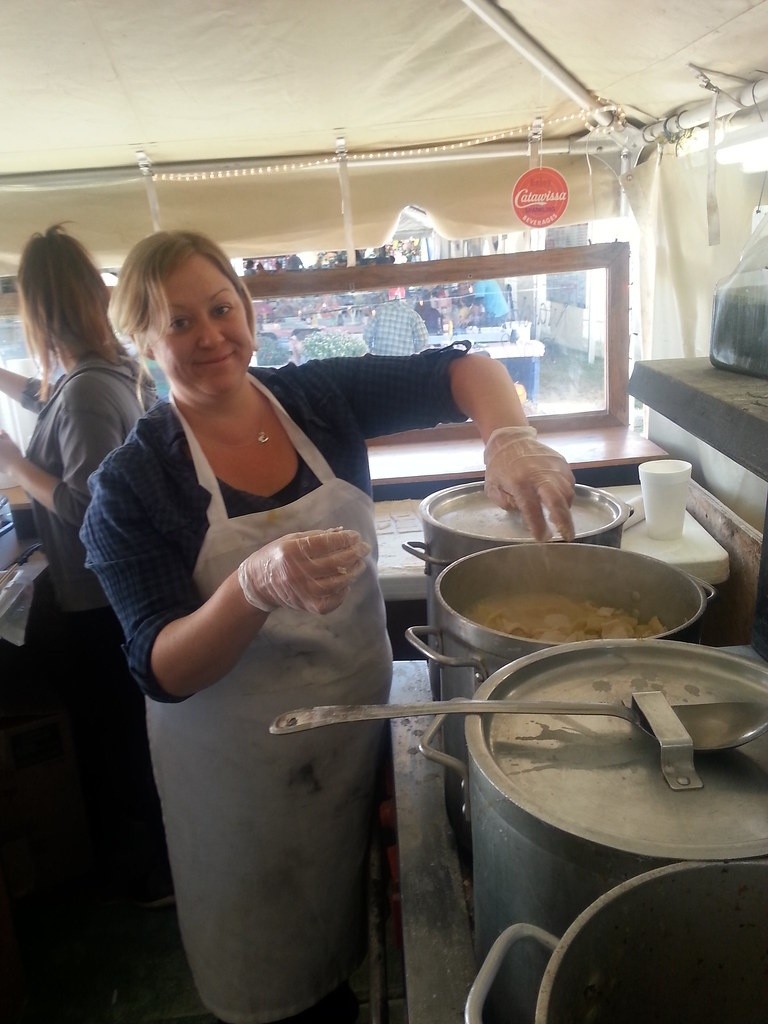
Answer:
(0, 222), (176, 913)
(240, 240), (515, 366)
(78, 231), (578, 1024)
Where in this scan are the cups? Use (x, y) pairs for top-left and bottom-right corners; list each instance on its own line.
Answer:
(639, 460), (693, 541)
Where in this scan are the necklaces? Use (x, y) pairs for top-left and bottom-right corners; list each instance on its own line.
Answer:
(196, 397), (269, 448)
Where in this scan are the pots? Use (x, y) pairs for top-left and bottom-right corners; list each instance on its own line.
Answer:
(407, 544), (715, 852)
(404, 479), (634, 703)
(464, 861), (768, 1024)
(421, 638), (768, 1024)
